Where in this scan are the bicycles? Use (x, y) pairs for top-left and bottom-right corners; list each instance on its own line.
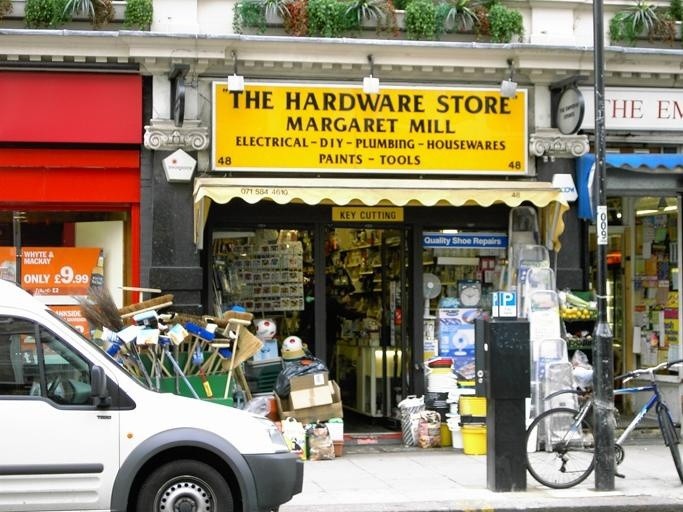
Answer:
(525, 358), (683, 489)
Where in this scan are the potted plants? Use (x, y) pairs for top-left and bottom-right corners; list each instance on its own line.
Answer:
(606, 3), (683, 44)
(1, 3), (153, 31)
(231, 0), (529, 43)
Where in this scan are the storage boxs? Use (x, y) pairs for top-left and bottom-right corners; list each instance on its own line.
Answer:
(436, 395), (488, 454)
(631, 372), (682, 428)
(273, 370), (345, 423)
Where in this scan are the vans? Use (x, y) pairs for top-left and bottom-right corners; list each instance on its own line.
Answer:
(0, 278), (304, 512)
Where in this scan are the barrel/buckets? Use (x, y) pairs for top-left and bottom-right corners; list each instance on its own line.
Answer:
(425, 356), (487, 454)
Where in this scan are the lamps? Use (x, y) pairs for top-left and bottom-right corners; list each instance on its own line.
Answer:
(362, 53), (380, 97)
(226, 48), (246, 94)
(499, 57), (518, 100)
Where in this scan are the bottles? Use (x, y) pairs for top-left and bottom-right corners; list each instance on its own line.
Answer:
(87, 249), (103, 299)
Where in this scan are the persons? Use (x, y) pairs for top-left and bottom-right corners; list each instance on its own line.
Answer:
(304, 278), (367, 372)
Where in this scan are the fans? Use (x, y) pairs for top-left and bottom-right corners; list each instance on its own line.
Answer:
(421, 272), (442, 320)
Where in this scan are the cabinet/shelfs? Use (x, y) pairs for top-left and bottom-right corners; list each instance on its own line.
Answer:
(604, 222), (682, 413)
(326, 237), (507, 416)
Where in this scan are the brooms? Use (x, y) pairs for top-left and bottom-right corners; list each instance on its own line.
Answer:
(75, 282), (262, 398)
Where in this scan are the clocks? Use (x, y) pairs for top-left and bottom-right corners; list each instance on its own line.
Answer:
(455, 280), (482, 307)
